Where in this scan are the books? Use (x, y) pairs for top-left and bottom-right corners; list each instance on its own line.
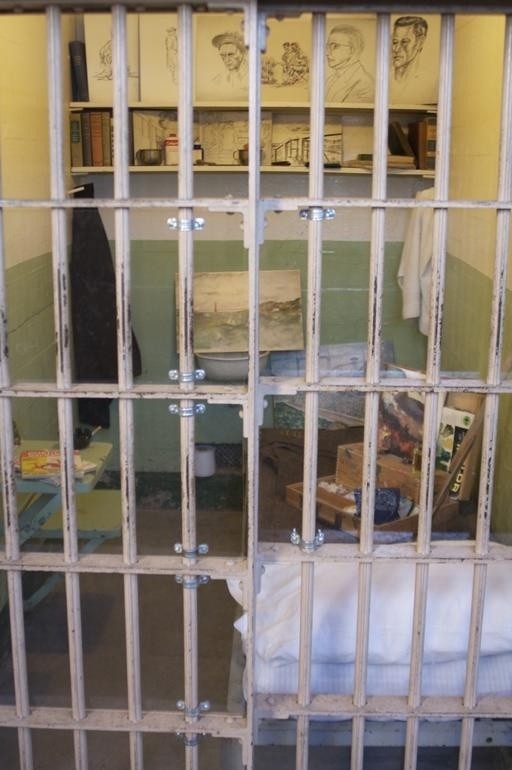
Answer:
(69, 113), (84, 167)
(20, 447), (85, 480)
(348, 111), (438, 172)
(81, 112), (93, 167)
(109, 117), (113, 165)
(70, 41), (86, 101)
(100, 111), (111, 166)
(91, 110), (104, 166)
(14, 457), (98, 487)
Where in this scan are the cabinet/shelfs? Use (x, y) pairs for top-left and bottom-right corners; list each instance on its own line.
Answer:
(71, 105), (441, 179)
(11, 438), (122, 614)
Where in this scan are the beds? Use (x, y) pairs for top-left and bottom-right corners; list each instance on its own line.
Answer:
(229, 542), (512, 769)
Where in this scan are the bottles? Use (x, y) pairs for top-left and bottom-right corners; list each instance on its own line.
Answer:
(165, 134), (178, 167)
(192, 144), (204, 165)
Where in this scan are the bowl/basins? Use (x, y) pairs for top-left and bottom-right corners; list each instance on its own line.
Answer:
(239, 150), (261, 166)
(135, 149), (163, 165)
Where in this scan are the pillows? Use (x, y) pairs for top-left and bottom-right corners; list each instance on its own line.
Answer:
(264, 559), (508, 665)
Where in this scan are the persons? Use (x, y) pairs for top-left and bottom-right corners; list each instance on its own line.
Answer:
(280, 43), (308, 80)
(276, 40), (289, 67)
(210, 30), (247, 92)
(389, 16), (429, 83)
(324, 23), (375, 103)
